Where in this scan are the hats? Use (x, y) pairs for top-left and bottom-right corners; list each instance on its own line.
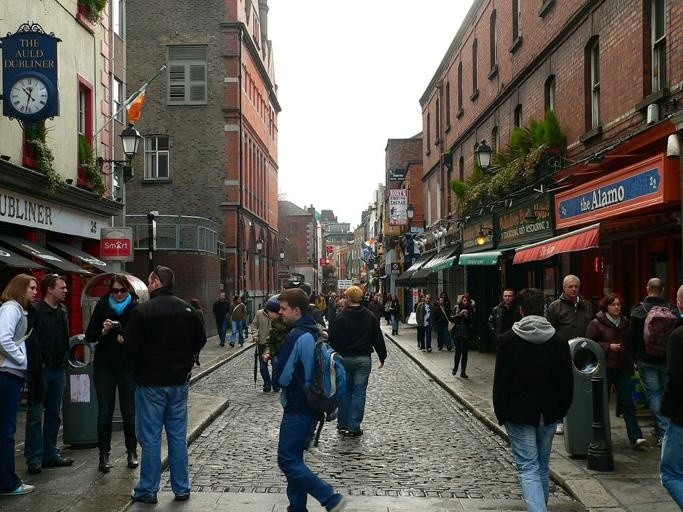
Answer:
(265, 292), (281, 312)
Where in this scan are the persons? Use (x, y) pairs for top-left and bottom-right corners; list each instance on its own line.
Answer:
(585, 293), (648, 448)
(657, 284), (683, 511)
(546, 274), (595, 436)
(490, 288), (522, 361)
(328, 286), (388, 437)
(85, 273), (141, 472)
(23, 273), (75, 476)
(0, 273), (37, 498)
(213, 291), (247, 347)
(118, 265), (207, 504)
(309, 290), (347, 328)
(362, 291), (401, 336)
(413, 291), (476, 379)
(250, 282), (327, 391)
(270, 287), (345, 512)
(189, 298), (207, 367)
(492, 287), (575, 512)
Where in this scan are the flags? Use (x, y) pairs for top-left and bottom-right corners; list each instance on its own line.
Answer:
(122, 66), (166, 126)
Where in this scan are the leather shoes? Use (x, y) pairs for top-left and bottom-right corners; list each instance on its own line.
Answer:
(174, 491), (189, 502)
(128, 450), (138, 469)
(263, 384), (269, 391)
(195, 358), (201, 367)
(131, 487), (158, 505)
(452, 368), (456, 375)
(460, 373), (469, 380)
(392, 332), (393, 334)
(100, 452), (109, 472)
(273, 387), (280, 392)
(396, 332), (398, 334)
(220, 342), (223, 346)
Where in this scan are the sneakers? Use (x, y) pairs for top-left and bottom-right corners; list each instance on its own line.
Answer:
(240, 342), (243, 345)
(338, 427), (345, 432)
(422, 349), (427, 352)
(230, 341), (234, 346)
(349, 428), (363, 438)
(556, 423), (564, 435)
(0, 484), (37, 496)
(42, 455), (75, 467)
(325, 493), (344, 512)
(631, 437), (647, 450)
(26, 458), (42, 477)
(428, 348), (432, 352)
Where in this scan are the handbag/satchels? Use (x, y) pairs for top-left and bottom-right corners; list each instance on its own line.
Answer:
(448, 305), (459, 332)
(439, 304), (450, 324)
(229, 303), (243, 322)
(0, 324), (35, 368)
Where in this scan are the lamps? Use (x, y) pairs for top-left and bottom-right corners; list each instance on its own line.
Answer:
(474, 224), (492, 246)
(99, 123), (143, 167)
(406, 204), (413, 232)
(249, 236), (264, 254)
(474, 139), (496, 175)
(277, 248), (285, 262)
(524, 207), (549, 219)
(373, 259), (379, 271)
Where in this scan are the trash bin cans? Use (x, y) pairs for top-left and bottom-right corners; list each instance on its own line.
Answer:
(564, 337), (611, 456)
(63, 334), (99, 446)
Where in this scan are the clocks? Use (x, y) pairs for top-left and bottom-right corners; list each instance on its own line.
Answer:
(9, 73), (55, 118)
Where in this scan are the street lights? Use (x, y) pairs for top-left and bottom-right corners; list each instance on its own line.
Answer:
(405, 206), (414, 220)
(278, 248), (285, 259)
(116, 123), (143, 159)
(372, 261), (377, 269)
(473, 139), (492, 170)
(255, 237), (263, 252)
(475, 231), (485, 245)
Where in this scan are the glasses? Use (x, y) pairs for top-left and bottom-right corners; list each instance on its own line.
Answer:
(153, 264), (165, 285)
(111, 287), (128, 295)
(42, 272), (63, 291)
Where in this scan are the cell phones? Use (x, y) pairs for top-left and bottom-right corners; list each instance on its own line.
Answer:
(110, 320), (120, 328)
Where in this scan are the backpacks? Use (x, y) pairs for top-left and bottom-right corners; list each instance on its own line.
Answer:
(639, 300), (678, 359)
(294, 326), (347, 448)
